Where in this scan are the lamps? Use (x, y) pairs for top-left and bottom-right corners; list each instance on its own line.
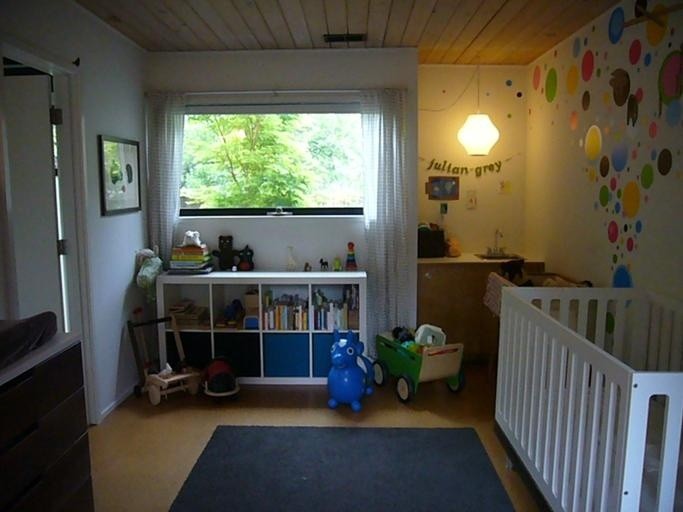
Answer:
(457, 113), (499, 157)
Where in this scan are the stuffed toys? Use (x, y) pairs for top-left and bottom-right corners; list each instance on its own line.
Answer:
(212, 235), (237, 270)
(235, 245), (254, 271)
(182, 229), (202, 245)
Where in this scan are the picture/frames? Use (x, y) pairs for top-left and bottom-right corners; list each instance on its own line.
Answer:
(97, 134), (142, 216)
(428, 176), (459, 201)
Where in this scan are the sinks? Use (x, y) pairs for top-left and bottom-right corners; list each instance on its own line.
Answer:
(481, 255), (519, 260)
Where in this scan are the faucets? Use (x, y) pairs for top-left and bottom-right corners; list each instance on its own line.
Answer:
(486, 228), (506, 255)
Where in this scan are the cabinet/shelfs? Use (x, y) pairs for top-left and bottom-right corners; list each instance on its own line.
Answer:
(417, 251), (545, 355)
(0, 332), (95, 512)
(155, 269), (369, 386)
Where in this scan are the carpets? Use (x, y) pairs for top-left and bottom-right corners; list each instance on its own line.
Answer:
(169, 424), (518, 512)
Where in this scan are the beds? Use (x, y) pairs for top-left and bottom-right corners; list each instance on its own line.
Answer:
(492, 286), (683, 512)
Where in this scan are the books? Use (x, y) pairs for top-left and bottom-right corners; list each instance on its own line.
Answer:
(167, 299), (209, 330)
(262, 290), (309, 331)
(311, 284), (359, 332)
(168, 245), (212, 274)
